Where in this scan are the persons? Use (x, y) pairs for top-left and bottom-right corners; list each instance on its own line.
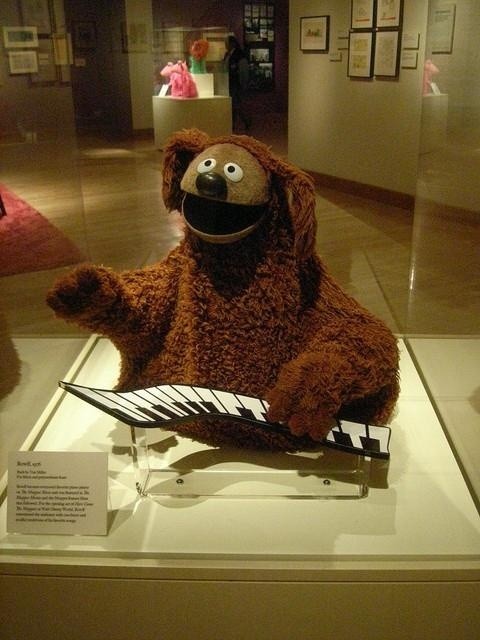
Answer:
(223, 34), (254, 128)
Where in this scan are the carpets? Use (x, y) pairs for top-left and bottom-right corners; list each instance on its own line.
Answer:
(0, 184), (85, 276)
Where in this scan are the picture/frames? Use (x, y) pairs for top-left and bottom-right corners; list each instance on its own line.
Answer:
(299, 1), (454, 79)
(1, 0), (99, 87)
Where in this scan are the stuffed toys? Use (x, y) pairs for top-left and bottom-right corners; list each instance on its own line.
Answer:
(159, 61), (199, 98)
(44, 126), (400, 451)
